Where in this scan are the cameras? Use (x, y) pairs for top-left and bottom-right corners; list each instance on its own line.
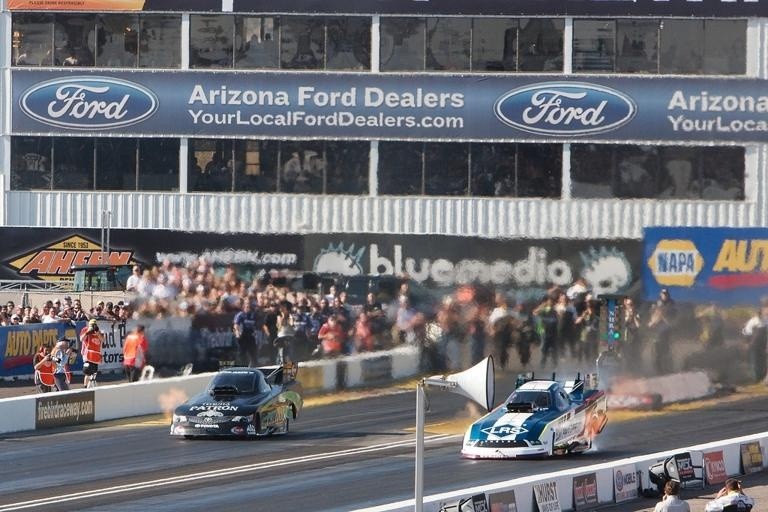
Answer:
(48, 355), (61, 364)
(90, 324), (99, 333)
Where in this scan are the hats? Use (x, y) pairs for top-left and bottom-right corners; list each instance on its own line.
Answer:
(88, 318), (97, 325)
(1, 295), (114, 321)
(58, 336), (71, 343)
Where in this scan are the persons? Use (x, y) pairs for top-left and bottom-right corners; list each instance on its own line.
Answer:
(706, 478), (754, 512)
(619, 288), (766, 384)
(1, 297), (148, 391)
(127, 256), (599, 374)
(654, 481), (690, 512)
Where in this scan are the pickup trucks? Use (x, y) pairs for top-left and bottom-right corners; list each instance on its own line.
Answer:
(301, 274), (438, 316)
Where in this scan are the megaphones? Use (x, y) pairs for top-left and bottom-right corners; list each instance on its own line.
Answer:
(423, 354), (497, 412)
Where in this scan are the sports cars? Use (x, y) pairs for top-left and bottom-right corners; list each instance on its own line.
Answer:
(462, 370), (611, 464)
(170, 360), (302, 443)
